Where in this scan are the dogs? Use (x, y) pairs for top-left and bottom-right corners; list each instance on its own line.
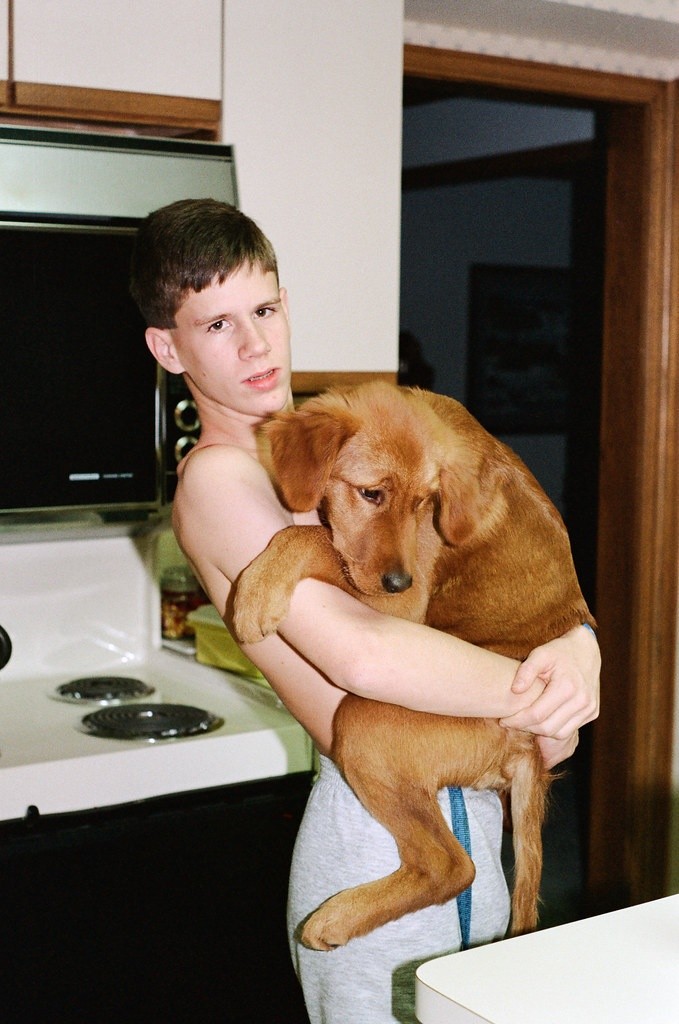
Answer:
(230, 381), (601, 953)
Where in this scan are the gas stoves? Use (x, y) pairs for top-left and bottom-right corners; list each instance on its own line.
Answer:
(44, 674), (224, 744)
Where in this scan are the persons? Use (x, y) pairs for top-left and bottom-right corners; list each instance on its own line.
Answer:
(125, 197), (601, 1024)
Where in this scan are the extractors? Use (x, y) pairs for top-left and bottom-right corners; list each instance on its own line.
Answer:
(0, 125), (241, 541)
